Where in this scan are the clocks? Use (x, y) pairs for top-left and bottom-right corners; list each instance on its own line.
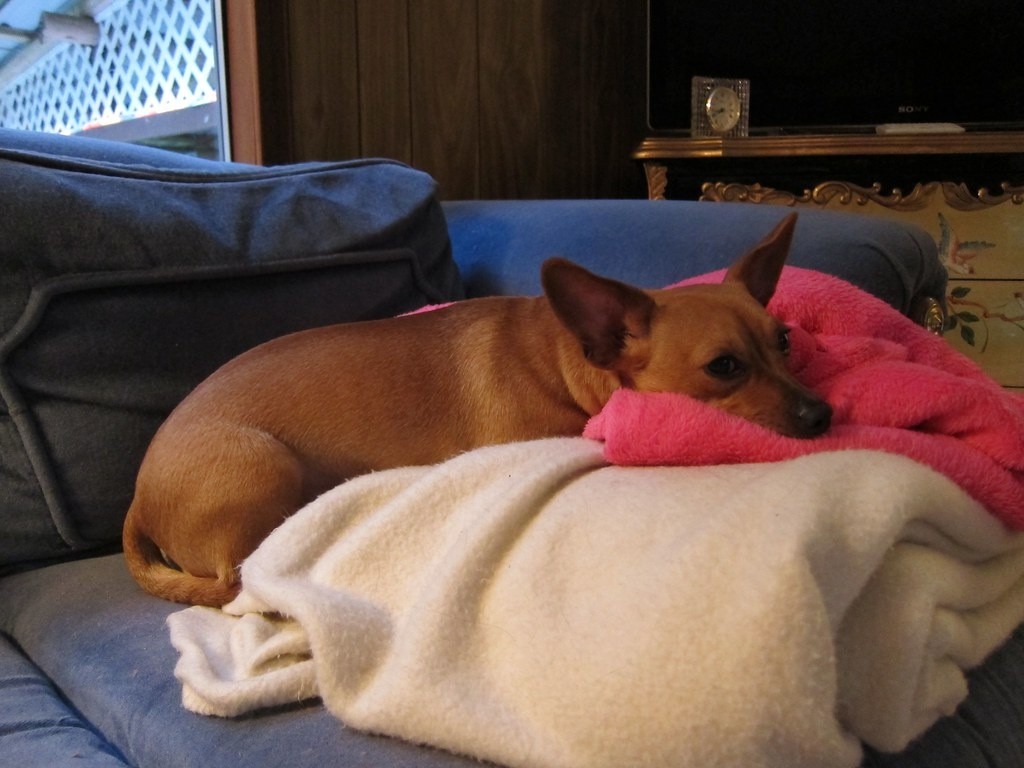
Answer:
(688, 76), (752, 139)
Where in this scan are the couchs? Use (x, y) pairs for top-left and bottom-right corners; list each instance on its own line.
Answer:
(1, 127), (1024, 768)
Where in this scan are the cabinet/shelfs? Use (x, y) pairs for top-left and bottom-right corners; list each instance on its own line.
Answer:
(634, 136), (1024, 399)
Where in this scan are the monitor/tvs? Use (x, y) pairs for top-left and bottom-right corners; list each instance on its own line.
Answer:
(646, 0), (1024, 132)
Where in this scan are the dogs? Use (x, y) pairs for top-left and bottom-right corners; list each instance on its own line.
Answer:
(121, 214), (833, 609)
